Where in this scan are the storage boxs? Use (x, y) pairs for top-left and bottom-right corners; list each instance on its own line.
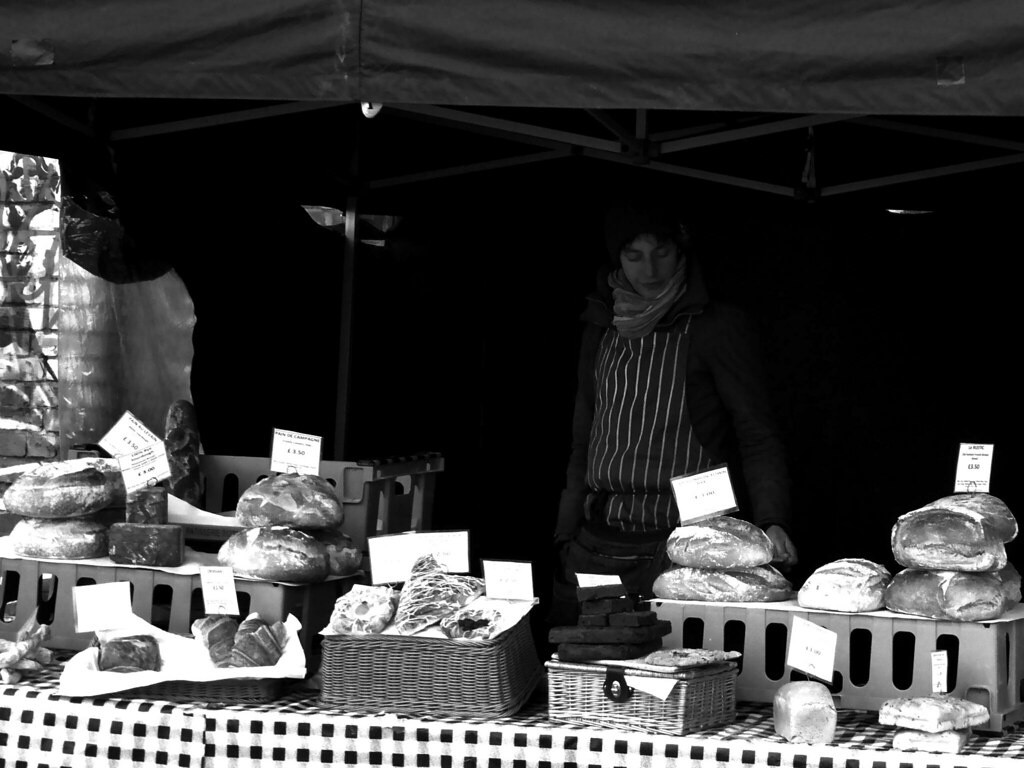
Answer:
(645, 590), (1024, 732)
(0, 536), (365, 672)
(199, 454), (444, 553)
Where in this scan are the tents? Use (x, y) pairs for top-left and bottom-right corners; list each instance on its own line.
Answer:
(0, 0), (1024, 461)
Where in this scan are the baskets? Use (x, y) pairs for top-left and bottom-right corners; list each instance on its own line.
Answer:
(111, 666), (287, 704)
(543, 653), (739, 736)
(316, 607), (544, 719)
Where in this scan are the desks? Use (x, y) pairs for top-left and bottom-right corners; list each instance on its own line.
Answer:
(0, 662), (1024, 768)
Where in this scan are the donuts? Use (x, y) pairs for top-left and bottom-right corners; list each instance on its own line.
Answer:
(330, 586), (396, 637)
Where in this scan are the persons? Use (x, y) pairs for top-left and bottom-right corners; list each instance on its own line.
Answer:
(547, 205), (799, 656)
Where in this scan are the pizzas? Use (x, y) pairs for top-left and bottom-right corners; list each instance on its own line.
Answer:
(644, 648), (740, 667)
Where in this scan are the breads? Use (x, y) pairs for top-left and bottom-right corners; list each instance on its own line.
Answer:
(192, 612), (287, 669)
(218, 472), (363, 584)
(884, 493), (1022, 622)
(3, 466), (114, 560)
(651, 515), (792, 604)
(548, 587), (673, 660)
(798, 558), (890, 613)
(100, 635), (160, 673)
(0, 625), (54, 684)
(876, 696), (990, 754)
(771, 681), (837, 744)
